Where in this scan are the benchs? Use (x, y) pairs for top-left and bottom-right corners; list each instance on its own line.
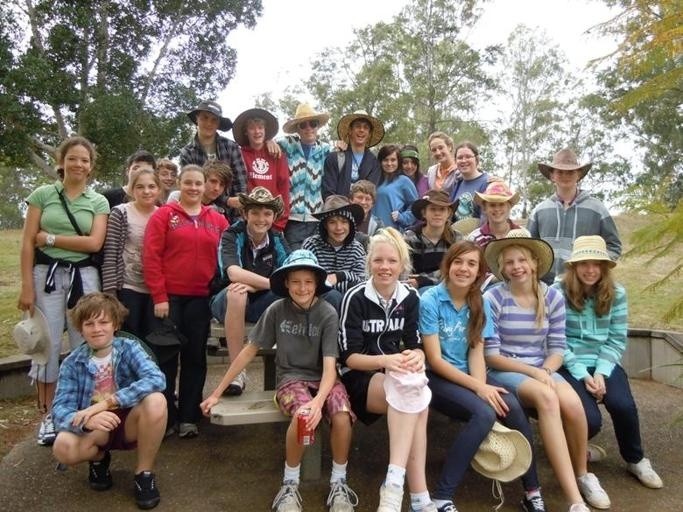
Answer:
(211, 316), (277, 391)
(210, 391), (321, 481)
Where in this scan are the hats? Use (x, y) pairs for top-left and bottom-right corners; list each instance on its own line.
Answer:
(145, 318), (186, 361)
(412, 148), (616, 279)
(187, 101), (384, 297)
(470, 421), (533, 483)
(383, 371), (432, 414)
(13, 304), (51, 365)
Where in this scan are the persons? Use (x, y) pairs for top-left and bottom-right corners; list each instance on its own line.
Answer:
(483, 228), (612, 512)
(50, 291), (169, 511)
(100, 102), (621, 440)
(197, 247), (358, 512)
(548, 233), (663, 491)
(337, 225), (436, 512)
(19, 135), (110, 447)
(418, 240), (547, 512)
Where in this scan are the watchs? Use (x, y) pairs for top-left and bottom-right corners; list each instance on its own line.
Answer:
(45, 233), (56, 247)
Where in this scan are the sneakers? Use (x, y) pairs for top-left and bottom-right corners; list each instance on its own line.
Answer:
(177, 422), (198, 439)
(88, 449), (114, 490)
(223, 367), (247, 395)
(132, 468), (159, 509)
(37, 414), (57, 445)
